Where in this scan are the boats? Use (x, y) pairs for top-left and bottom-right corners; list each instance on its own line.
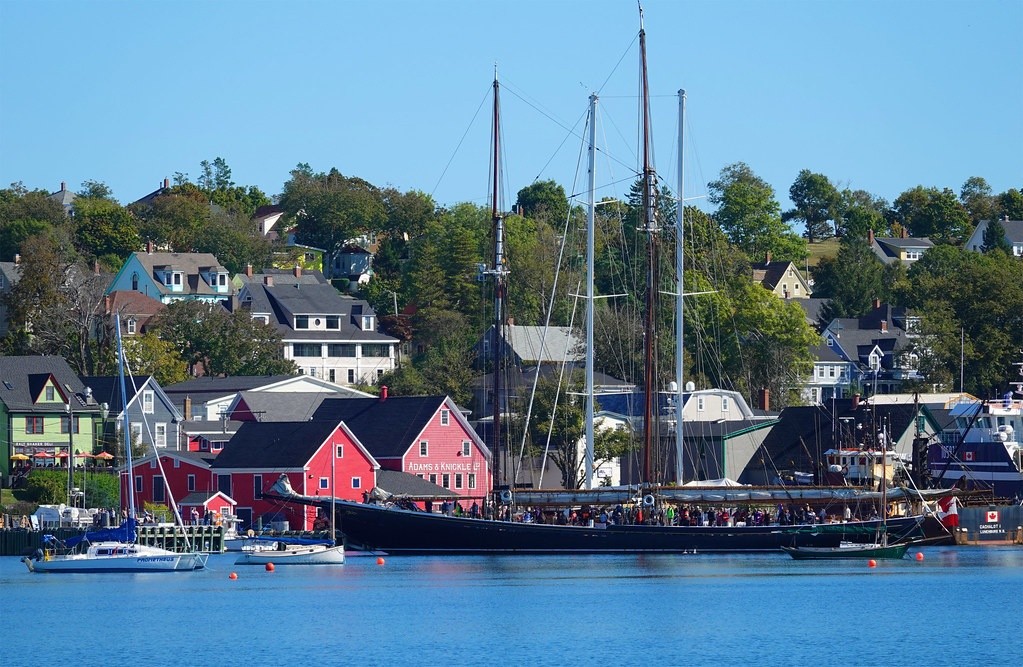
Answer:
(781, 424), (915, 561)
(234, 441), (346, 565)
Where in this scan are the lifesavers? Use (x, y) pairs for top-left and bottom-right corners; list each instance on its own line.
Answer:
(501, 490), (512, 500)
(643, 495), (655, 505)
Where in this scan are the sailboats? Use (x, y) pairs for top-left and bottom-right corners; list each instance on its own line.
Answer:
(20, 308), (210, 570)
(254, 0), (986, 558)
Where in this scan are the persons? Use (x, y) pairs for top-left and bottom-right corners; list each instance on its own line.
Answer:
(13, 461), (112, 469)
(439, 502), (878, 527)
(101, 506), (200, 525)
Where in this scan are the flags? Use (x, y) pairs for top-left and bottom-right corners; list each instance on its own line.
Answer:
(963, 451), (975, 461)
(985, 511), (1000, 522)
(938, 488), (959, 528)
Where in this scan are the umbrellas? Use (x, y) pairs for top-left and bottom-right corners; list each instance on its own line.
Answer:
(74, 450), (95, 464)
(10, 453), (30, 469)
(94, 450), (114, 466)
(56, 451), (67, 462)
(34, 451), (54, 465)
